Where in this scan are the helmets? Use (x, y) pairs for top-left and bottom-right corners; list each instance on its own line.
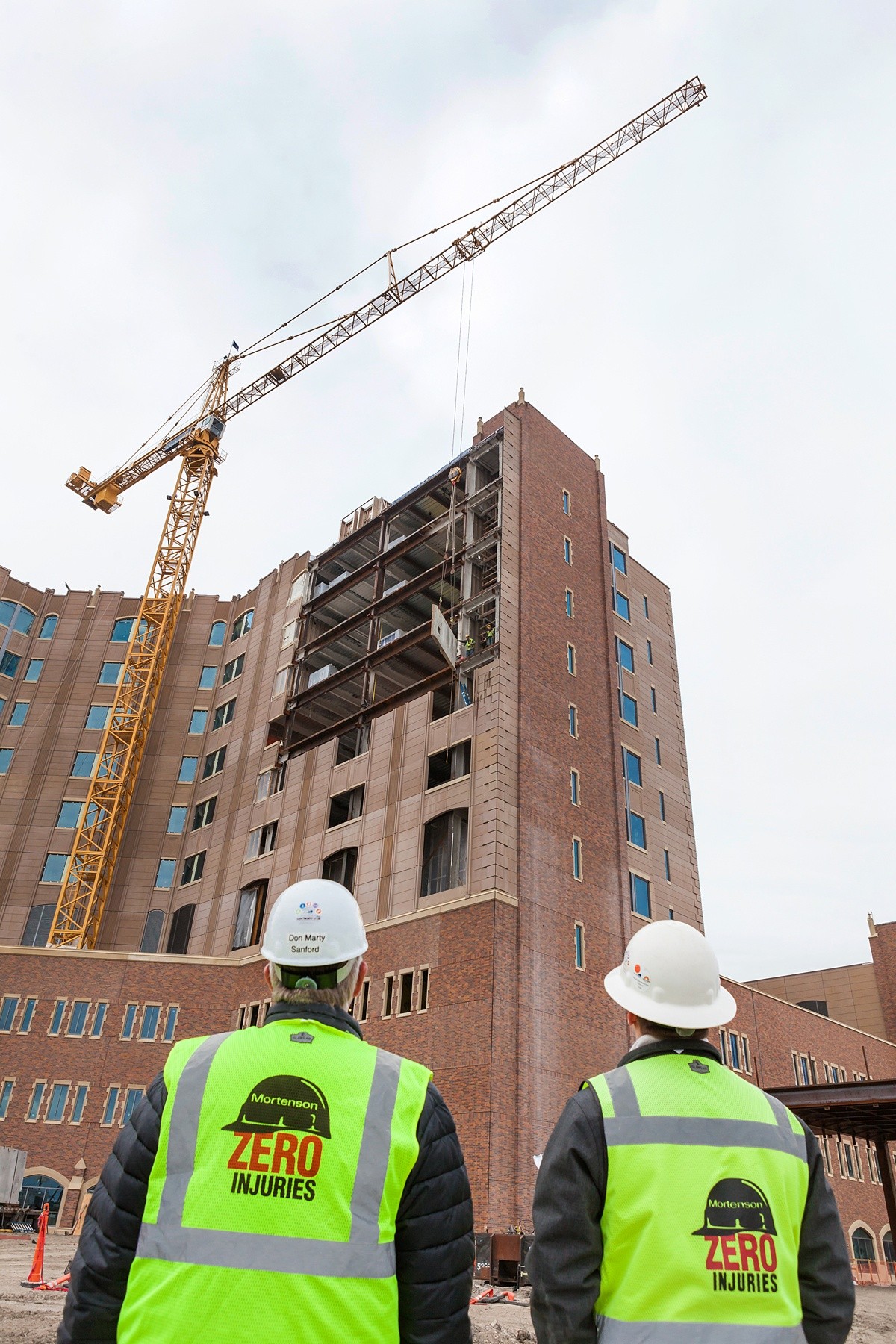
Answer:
(603, 920), (737, 1029)
(261, 878), (369, 967)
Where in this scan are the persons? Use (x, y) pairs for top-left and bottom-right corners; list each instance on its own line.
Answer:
(526, 919), (857, 1343)
(53, 878), (476, 1343)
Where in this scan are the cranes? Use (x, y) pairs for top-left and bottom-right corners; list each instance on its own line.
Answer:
(43, 71), (709, 959)
